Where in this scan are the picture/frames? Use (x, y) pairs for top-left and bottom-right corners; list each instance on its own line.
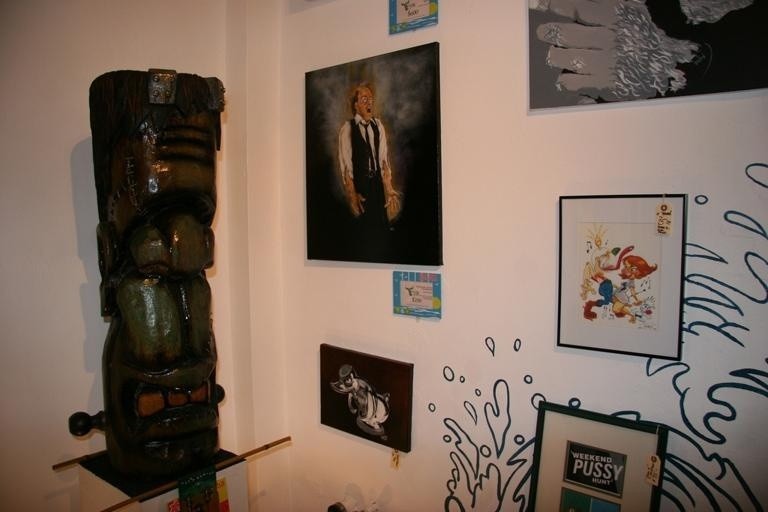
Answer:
(392, 269), (442, 320)
(386, 0), (441, 37)
(319, 343), (415, 455)
(524, 399), (669, 512)
(524, 0), (767, 118)
(556, 193), (688, 363)
